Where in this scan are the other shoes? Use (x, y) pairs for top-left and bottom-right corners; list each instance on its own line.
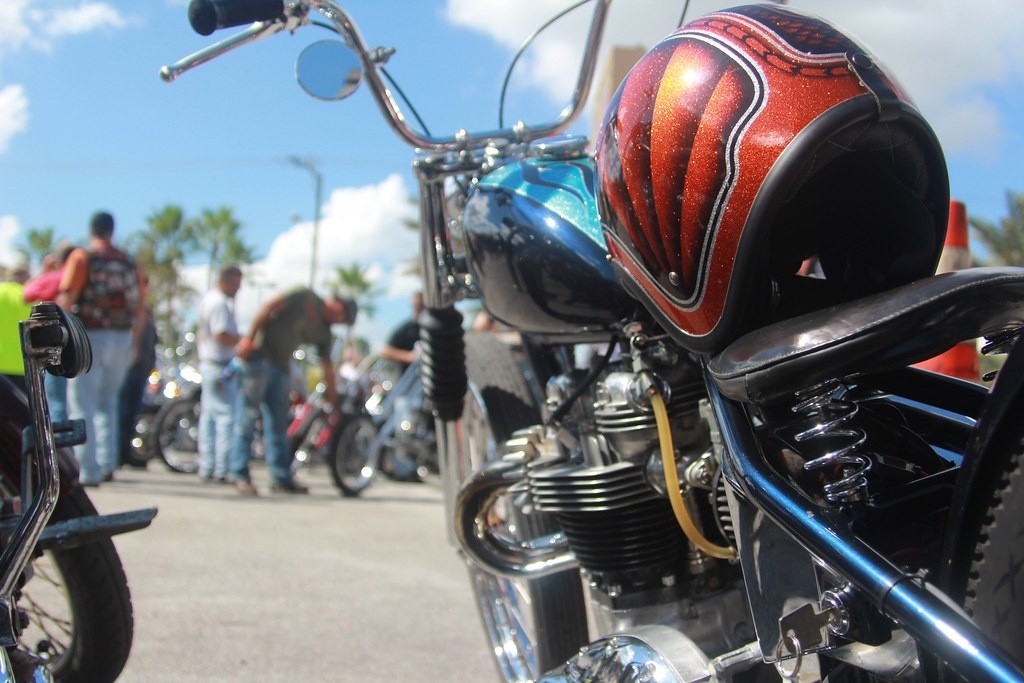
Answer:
(269, 478), (308, 495)
(232, 470), (257, 496)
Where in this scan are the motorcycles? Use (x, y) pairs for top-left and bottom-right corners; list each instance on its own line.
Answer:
(0, 278), (161, 683)
(154, 0), (1024, 683)
(121, 323), (438, 501)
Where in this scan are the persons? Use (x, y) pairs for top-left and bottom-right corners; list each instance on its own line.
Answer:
(117, 272), (158, 469)
(23, 239), (75, 454)
(0, 247), (37, 375)
(229, 285), (357, 494)
(380, 292), (439, 483)
(338, 337), (371, 393)
(59, 210), (146, 486)
(195, 267), (242, 485)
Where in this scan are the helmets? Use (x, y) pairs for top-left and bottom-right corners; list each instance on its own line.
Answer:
(596, 2), (950, 356)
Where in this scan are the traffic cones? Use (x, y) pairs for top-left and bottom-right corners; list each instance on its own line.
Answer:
(908, 200), (982, 384)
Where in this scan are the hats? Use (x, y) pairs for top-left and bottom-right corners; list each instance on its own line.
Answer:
(340, 297), (357, 325)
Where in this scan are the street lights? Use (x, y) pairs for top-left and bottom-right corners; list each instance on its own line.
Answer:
(290, 152), (325, 400)
